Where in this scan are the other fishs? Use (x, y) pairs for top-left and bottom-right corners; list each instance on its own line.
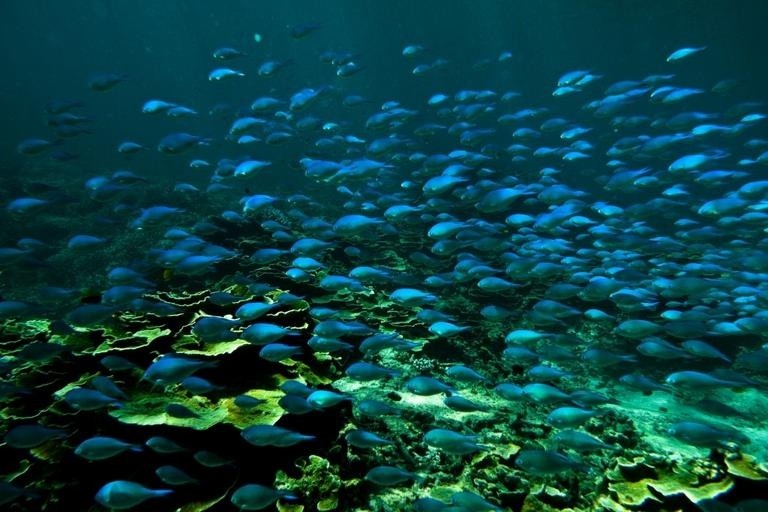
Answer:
(0, 18), (768, 512)
